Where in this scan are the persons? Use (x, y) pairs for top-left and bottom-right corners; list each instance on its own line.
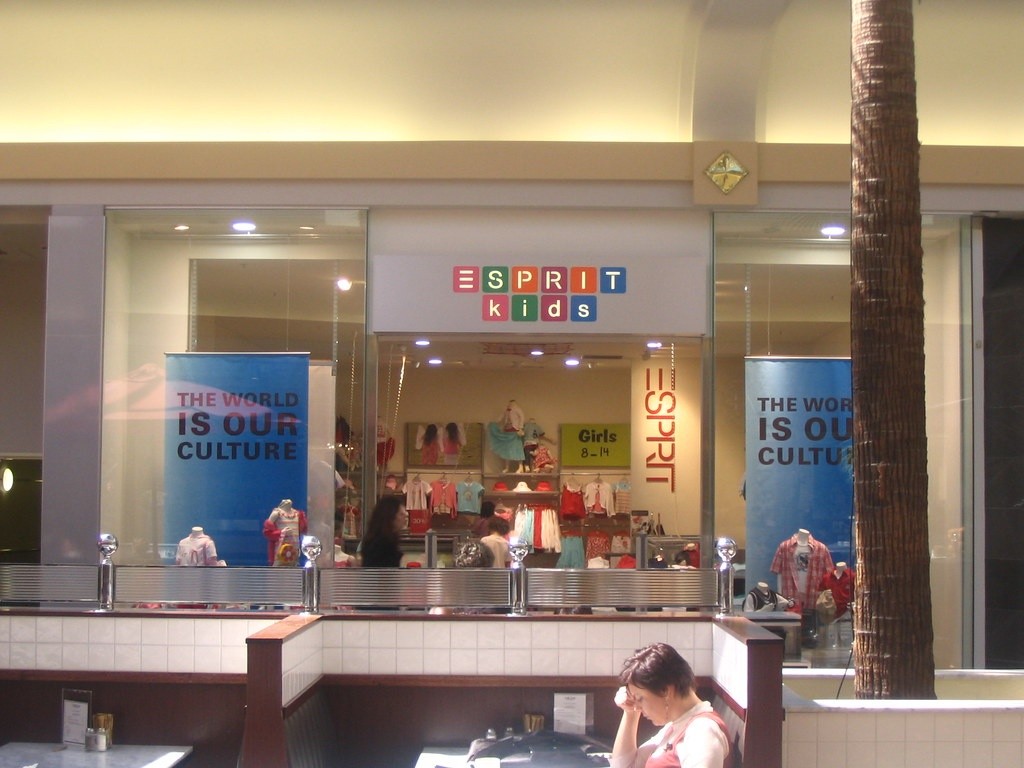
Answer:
(609, 644), (734, 768)
(333, 545), (355, 610)
(454, 539), (506, 614)
(742, 529), (854, 644)
(354, 496), (408, 610)
(256, 499), (308, 610)
(176, 527), (218, 609)
(480, 518), (511, 568)
(488, 400), (557, 473)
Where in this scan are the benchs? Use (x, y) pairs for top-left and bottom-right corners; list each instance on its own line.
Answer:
(283, 673), (343, 768)
(738, 611), (802, 659)
(710, 677), (746, 768)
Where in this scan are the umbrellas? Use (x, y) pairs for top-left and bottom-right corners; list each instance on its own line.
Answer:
(47, 363), (301, 560)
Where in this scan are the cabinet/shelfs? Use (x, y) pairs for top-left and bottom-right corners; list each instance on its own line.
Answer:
(335, 470), (405, 496)
(484, 472), (561, 500)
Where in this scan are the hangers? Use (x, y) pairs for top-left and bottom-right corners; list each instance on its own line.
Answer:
(594, 472), (601, 483)
(439, 471), (447, 483)
(412, 471), (421, 486)
(567, 472), (580, 487)
(620, 473), (629, 484)
(464, 471), (473, 483)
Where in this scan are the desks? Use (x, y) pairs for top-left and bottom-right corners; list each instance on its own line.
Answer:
(0, 741), (194, 768)
(415, 746), (614, 768)
